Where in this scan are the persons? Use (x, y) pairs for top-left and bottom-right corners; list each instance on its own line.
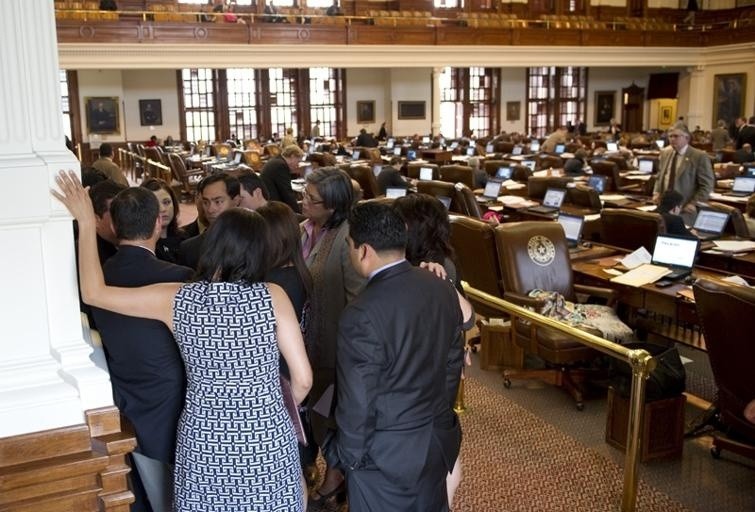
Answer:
(47, 165), (480, 512)
(197, 0), (346, 28)
(89, 144), (131, 188)
(143, 113), (755, 263)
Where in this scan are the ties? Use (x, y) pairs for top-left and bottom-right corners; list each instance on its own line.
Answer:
(666, 151), (679, 193)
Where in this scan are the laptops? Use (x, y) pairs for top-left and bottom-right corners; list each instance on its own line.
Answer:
(478, 180), (502, 202)
(529, 187), (568, 215)
(520, 160), (537, 173)
(206, 137), (755, 279)
(651, 232), (700, 279)
(689, 208), (732, 241)
(629, 158), (655, 176)
(385, 186), (409, 199)
(554, 144), (567, 156)
(722, 174), (755, 197)
(490, 166), (514, 182)
(418, 168), (434, 182)
(555, 212), (585, 248)
(435, 195), (453, 211)
(587, 174), (606, 194)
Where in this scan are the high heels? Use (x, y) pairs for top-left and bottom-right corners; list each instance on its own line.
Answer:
(304, 481), (347, 508)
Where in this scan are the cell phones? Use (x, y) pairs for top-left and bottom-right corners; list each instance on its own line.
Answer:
(656, 281), (673, 287)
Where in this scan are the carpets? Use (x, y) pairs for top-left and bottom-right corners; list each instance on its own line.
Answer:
(446, 377), (703, 512)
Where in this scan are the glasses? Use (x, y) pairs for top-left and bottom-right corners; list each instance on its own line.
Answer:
(301, 184), (325, 207)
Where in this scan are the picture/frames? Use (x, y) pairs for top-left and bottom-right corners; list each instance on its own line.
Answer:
(139, 99), (163, 126)
(398, 100), (426, 120)
(593, 89), (617, 127)
(711, 72), (749, 130)
(357, 100), (376, 124)
(506, 100), (521, 121)
(84, 95), (121, 136)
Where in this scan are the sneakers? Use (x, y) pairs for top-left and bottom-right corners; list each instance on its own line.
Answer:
(303, 459), (320, 485)
